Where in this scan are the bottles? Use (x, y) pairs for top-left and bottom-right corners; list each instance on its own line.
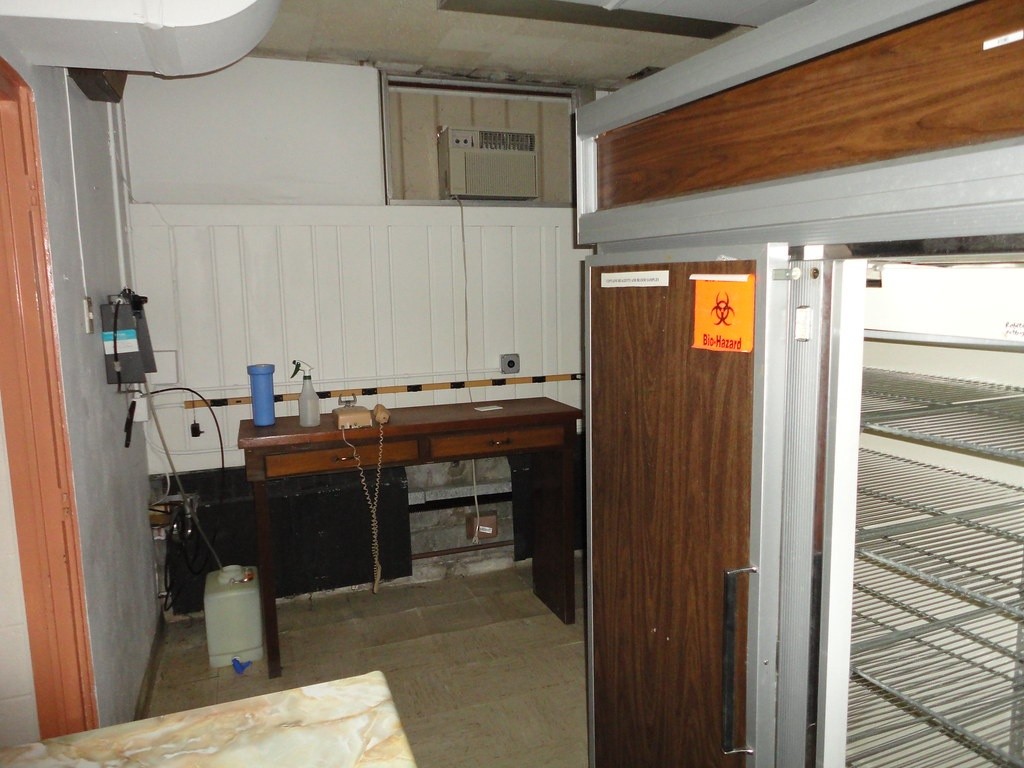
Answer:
(204, 564), (264, 668)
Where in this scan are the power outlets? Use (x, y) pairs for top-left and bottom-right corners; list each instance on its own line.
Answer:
(500, 353), (520, 374)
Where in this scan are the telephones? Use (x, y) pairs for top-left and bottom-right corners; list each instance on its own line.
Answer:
(332, 393), (390, 430)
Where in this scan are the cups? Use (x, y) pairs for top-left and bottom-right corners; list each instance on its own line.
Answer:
(247, 363), (275, 427)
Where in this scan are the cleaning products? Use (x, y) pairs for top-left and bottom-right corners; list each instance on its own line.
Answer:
(290, 359), (320, 427)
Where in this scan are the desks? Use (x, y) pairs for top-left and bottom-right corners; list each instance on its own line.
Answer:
(238, 395), (586, 679)
